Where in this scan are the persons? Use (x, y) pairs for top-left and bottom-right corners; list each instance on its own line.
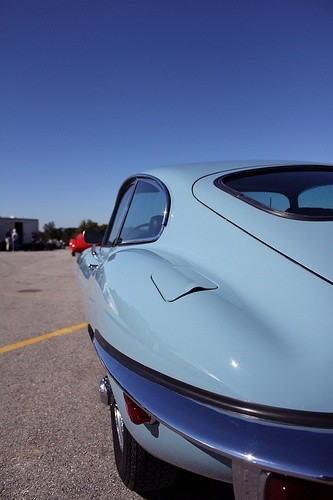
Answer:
(5, 229), (11, 251)
(10, 228), (19, 252)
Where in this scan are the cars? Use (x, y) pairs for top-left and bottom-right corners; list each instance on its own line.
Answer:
(77, 157), (332, 497)
(68, 234), (99, 257)
